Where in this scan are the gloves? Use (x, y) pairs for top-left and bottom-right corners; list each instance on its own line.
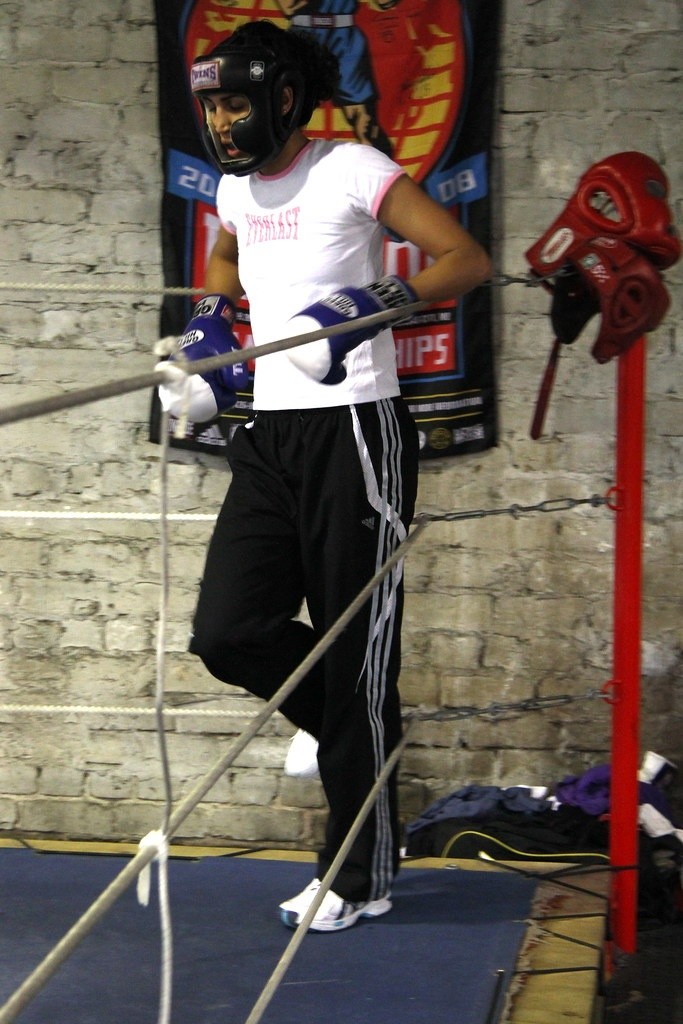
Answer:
(155, 294), (249, 423)
(284, 274), (416, 385)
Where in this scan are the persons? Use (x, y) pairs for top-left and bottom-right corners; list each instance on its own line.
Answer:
(154, 20), (492, 932)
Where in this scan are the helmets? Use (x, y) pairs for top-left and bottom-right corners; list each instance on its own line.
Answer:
(190, 32), (314, 177)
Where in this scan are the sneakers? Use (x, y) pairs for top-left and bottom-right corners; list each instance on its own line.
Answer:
(278, 877), (392, 932)
(284, 728), (319, 777)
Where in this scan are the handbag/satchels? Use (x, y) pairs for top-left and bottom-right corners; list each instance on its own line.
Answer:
(404, 784), (610, 862)
